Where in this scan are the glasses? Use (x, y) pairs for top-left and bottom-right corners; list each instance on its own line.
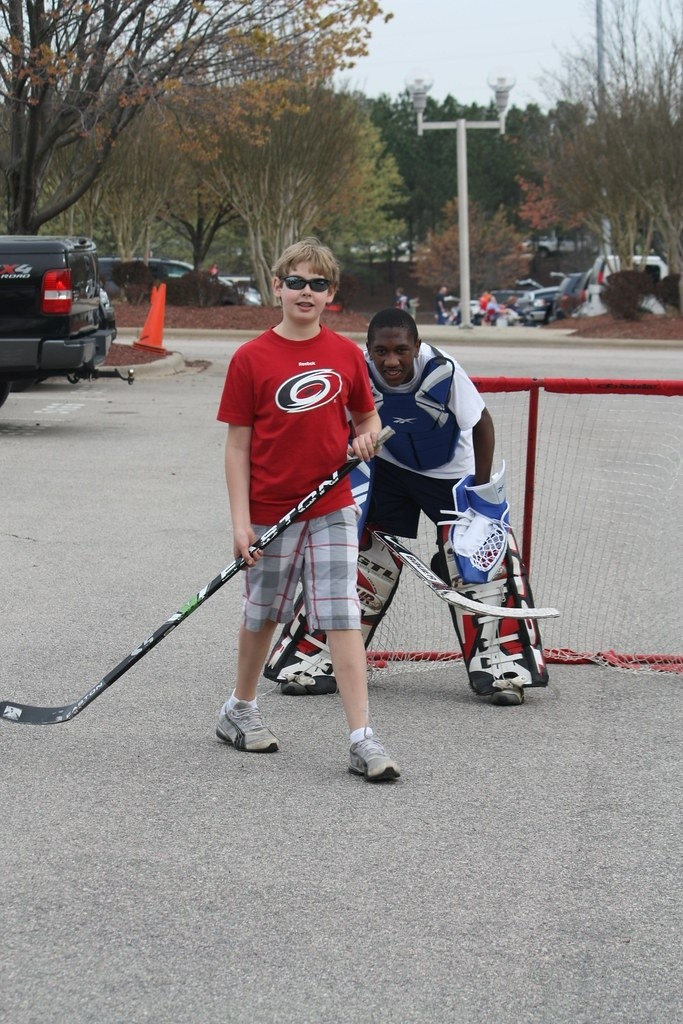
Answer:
(279, 275), (333, 293)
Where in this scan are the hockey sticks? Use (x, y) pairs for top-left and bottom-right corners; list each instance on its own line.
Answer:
(1, 425), (397, 725)
(364, 520), (561, 619)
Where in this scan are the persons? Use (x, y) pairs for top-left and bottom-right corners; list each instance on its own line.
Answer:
(395, 288), (411, 313)
(435, 286), (449, 325)
(480, 290), (500, 325)
(216, 238), (398, 775)
(280, 308), (526, 706)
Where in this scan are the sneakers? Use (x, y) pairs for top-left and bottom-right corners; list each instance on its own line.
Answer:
(348, 738), (400, 783)
(216, 700), (278, 753)
(281, 682), (308, 696)
(490, 686), (525, 705)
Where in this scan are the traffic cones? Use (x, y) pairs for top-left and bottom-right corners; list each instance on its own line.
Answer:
(134, 283), (171, 355)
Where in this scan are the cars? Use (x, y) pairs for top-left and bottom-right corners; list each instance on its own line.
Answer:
(485, 251), (670, 319)
(520, 229), (591, 258)
(96, 257), (264, 308)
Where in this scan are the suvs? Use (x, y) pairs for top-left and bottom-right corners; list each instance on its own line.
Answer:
(0, 234), (140, 407)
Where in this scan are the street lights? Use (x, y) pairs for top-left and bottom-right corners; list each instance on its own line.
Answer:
(405, 69), (517, 331)
